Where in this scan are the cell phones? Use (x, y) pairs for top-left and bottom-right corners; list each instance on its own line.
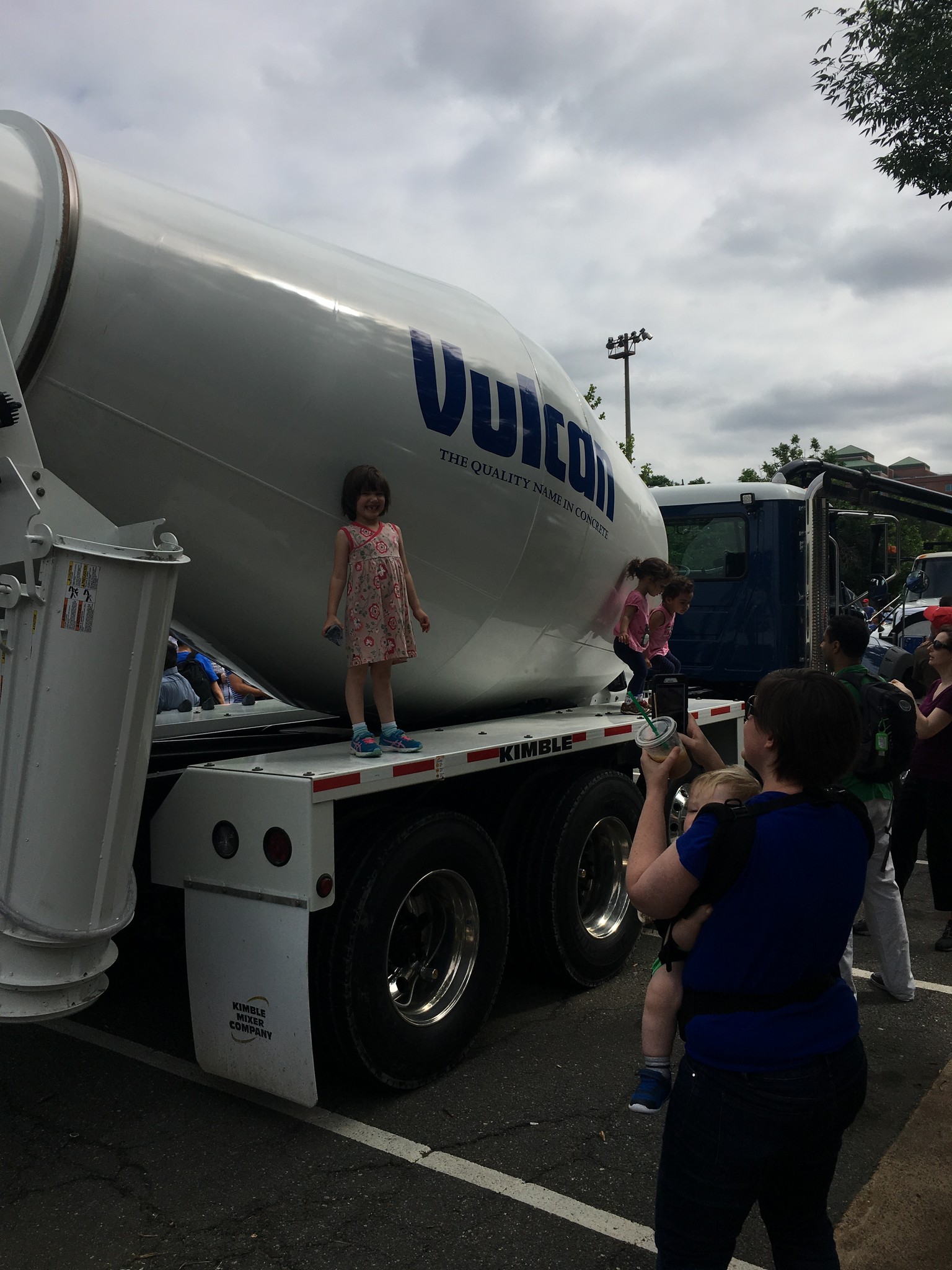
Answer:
(652, 672), (688, 748)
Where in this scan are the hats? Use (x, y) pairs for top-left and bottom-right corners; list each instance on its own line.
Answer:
(924, 606), (952, 630)
(863, 599), (869, 603)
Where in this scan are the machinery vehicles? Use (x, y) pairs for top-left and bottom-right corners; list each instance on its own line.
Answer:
(865, 541), (952, 687)
(0, 106), (929, 1112)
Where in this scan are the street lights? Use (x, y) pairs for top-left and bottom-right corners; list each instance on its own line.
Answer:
(606, 328), (653, 464)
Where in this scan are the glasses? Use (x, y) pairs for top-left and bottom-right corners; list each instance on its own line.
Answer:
(746, 695), (759, 720)
(931, 639), (950, 650)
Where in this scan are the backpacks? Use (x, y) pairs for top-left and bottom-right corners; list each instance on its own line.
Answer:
(836, 671), (917, 782)
(177, 652), (219, 705)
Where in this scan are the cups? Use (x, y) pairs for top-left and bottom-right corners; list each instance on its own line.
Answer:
(635, 715), (692, 780)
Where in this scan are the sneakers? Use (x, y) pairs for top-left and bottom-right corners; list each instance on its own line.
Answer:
(620, 702), (650, 714)
(628, 1068), (673, 1114)
(870, 971), (888, 991)
(639, 700), (652, 711)
(350, 731), (382, 757)
(853, 919), (871, 936)
(934, 919), (952, 951)
(379, 729), (422, 752)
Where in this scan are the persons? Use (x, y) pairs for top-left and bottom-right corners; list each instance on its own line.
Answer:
(151, 634), (275, 715)
(644, 575), (694, 712)
(626, 667), (875, 1270)
(816, 614), (918, 1006)
(629, 764), (763, 1114)
(613, 558), (674, 713)
(840, 566), (952, 952)
(320, 464), (431, 757)
